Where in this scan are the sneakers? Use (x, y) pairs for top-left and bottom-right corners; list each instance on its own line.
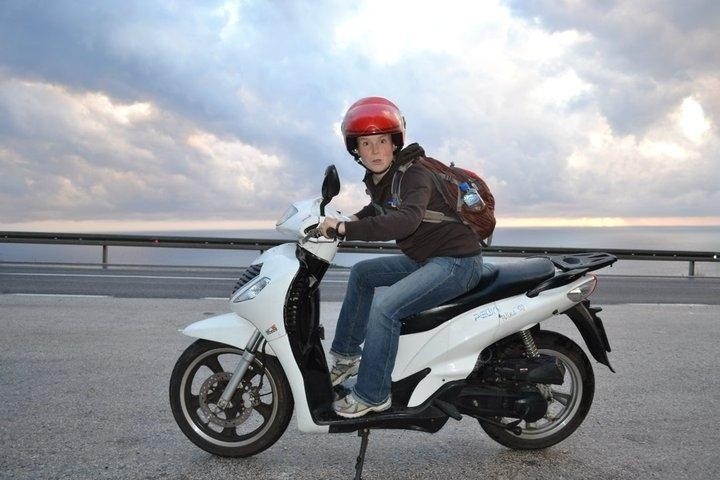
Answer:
(331, 391), (393, 419)
(329, 354), (363, 388)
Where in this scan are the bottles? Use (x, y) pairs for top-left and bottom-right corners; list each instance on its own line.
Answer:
(459, 182), (485, 209)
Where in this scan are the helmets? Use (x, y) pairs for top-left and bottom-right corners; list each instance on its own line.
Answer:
(340, 95), (406, 157)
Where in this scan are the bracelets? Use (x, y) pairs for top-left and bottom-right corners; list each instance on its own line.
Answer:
(334, 220), (342, 236)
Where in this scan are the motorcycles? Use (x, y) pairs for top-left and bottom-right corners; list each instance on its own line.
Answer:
(168, 164), (617, 480)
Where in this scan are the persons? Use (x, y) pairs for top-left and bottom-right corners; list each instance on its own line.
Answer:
(312, 96), (493, 426)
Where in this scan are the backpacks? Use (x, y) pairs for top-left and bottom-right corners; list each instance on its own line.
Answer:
(388, 154), (497, 248)
